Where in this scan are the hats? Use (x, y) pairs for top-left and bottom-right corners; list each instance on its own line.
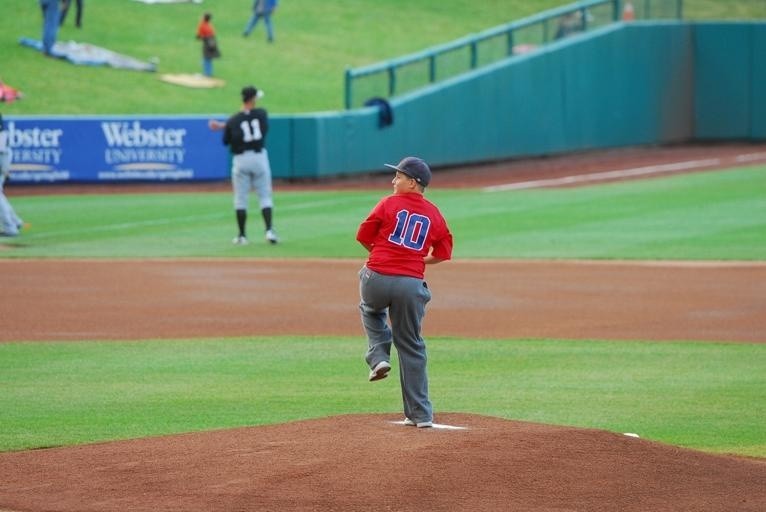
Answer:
(383, 156), (432, 186)
(242, 87), (265, 99)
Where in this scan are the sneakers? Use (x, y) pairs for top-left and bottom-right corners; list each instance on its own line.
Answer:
(404, 417), (432, 428)
(232, 236), (247, 245)
(265, 229), (277, 243)
(369, 360), (391, 381)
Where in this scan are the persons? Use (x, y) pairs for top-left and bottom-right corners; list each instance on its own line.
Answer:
(39, 1), (59, 58)
(59, 1), (82, 28)
(0, 109), (24, 239)
(207, 85), (278, 243)
(241, 0), (279, 43)
(196, 11), (216, 80)
(353, 155), (452, 427)
